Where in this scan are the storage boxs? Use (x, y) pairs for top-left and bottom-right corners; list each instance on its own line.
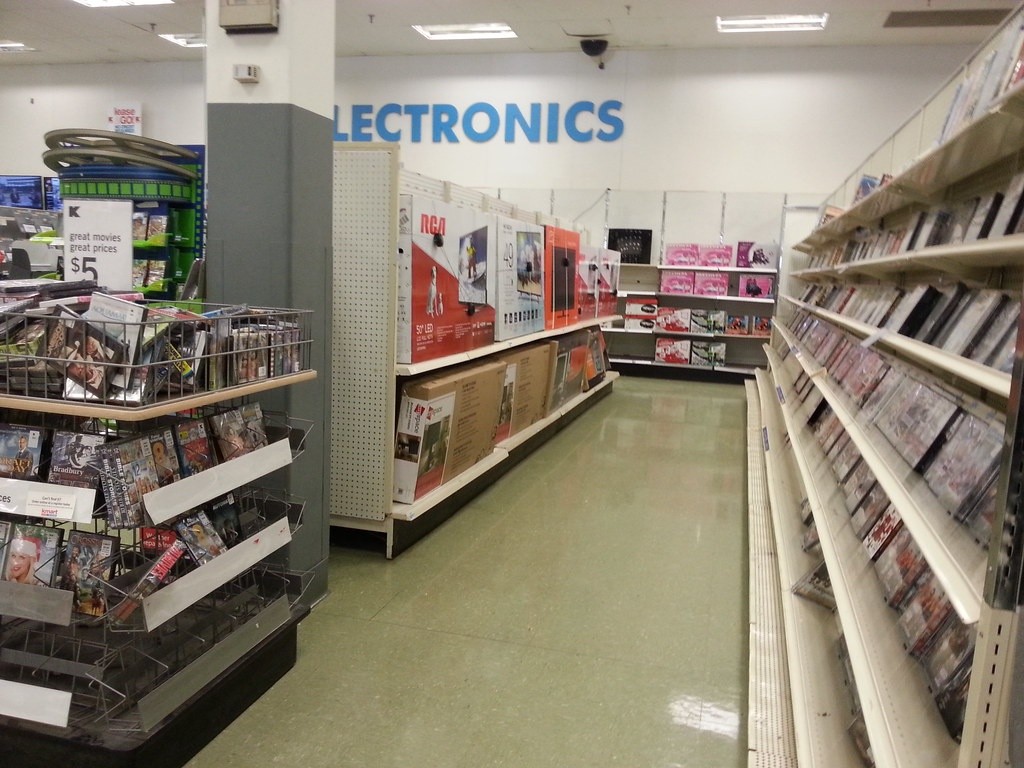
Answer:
(392, 187), (787, 510)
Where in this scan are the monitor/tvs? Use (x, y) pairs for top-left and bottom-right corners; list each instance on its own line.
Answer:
(0, 174), (64, 212)
(458, 226), (575, 318)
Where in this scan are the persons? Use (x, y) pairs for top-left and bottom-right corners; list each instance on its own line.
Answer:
(4, 535), (41, 585)
(11, 434), (34, 479)
(54, 338), (107, 386)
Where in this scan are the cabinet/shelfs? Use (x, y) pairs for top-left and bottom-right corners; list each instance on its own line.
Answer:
(593, 264), (779, 386)
(329, 138), (621, 557)
(743, 79), (1024, 768)
(0, 368), (311, 768)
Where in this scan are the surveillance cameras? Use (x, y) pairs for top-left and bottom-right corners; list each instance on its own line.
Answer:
(581, 39), (608, 57)
